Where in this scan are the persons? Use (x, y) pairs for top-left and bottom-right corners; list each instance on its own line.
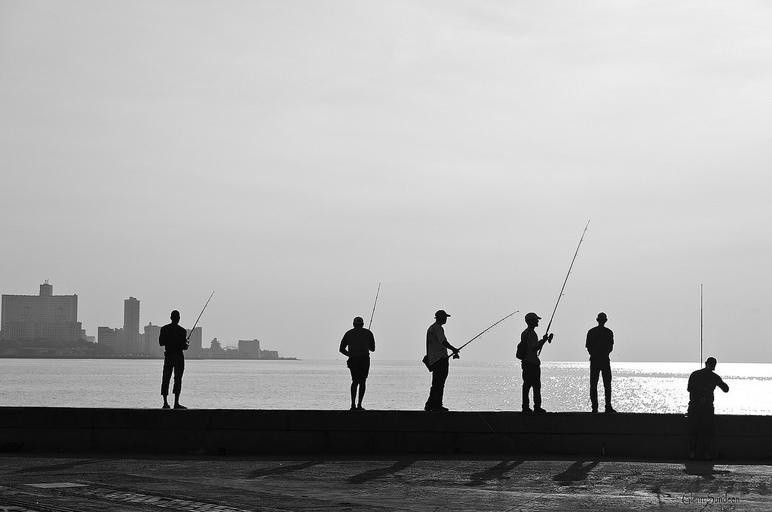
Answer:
(686, 356), (729, 415)
(338, 316), (375, 409)
(584, 311), (616, 412)
(423, 309), (461, 411)
(519, 312), (550, 414)
(158, 309), (190, 409)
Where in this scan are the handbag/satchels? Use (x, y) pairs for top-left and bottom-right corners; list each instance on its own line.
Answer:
(515, 341), (528, 359)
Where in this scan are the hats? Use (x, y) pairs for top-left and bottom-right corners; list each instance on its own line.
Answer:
(524, 312), (543, 322)
(434, 309), (452, 319)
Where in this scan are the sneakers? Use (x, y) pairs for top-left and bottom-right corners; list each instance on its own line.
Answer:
(521, 405), (547, 413)
(593, 407), (618, 414)
(425, 400), (448, 412)
(162, 404), (188, 409)
(350, 404), (365, 411)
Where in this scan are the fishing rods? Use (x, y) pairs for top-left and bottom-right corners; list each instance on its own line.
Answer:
(537, 220), (590, 357)
(447, 311), (519, 358)
(185, 291), (215, 349)
(700, 284), (703, 368)
(369, 283), (380, 330)
